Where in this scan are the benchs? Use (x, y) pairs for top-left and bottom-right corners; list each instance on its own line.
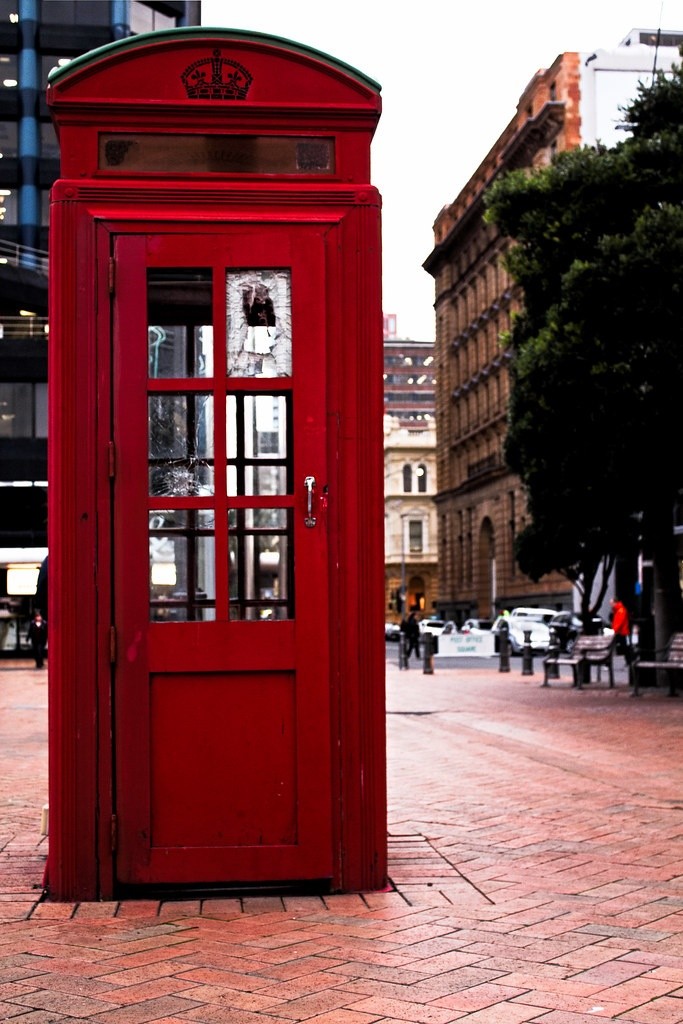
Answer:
(632, 632), (683, 697)
(543, 633), (617, 689)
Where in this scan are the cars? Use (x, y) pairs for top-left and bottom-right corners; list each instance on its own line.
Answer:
(460, 619), (493, 634)
(492, 615), (550, 654)
(385, 620), (402, 642)
(547, 610), (613, 653)
(418, 619), (447, 637)
(512, 607), (557, 623)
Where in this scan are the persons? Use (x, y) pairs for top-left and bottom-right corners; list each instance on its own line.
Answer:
(443, 625), (453, 634)
(26, 611), (48, 668)
(491, 610), (512, 653)
(406, 613), (423, 661)
(463, 622), (476, 633)
(610, 597), (634, 665)
(36, 556), (48, 622)
